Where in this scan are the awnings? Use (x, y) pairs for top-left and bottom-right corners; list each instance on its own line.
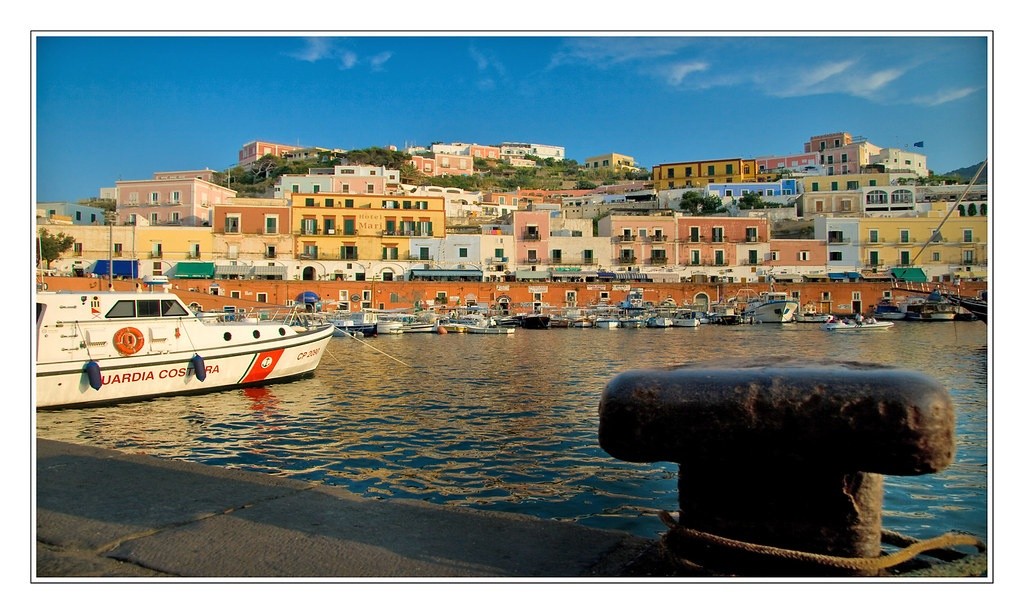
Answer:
(515, 271), (551, 279)
(299, 292), (319, 303)
(175, 262), (215, 279)
(215, 266), (254, 275)
(829, 272), (861, 280)
(549, 270), (582, 277)
(615, 273), (647, 280)
(413, 270), (482, 277)
(93, 260), (138, 278)
(253, 265), (285, 275)
(892, 268), (927, 283)
(597, 272), (615, 279)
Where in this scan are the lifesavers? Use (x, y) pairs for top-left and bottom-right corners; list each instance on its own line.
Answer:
(114, 328), (145, 354)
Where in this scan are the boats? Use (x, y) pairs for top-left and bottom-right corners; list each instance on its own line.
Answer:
(196, 291), (988, 335)
(826, 322), (895, 331)
(36, 222), (335, 411)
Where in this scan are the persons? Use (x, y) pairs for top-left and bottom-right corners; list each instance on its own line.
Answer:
(86, 272), (98, 278)
(843, 316), (850, 324)
(855, 311), (876, 326)
(826, 317), (842, 323)
